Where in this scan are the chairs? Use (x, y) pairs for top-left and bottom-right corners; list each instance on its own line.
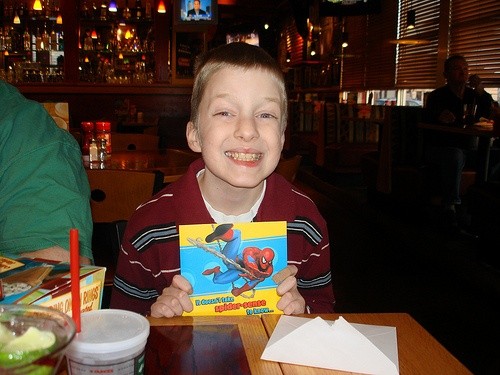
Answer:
(111, 133), (159, 154)
(86, 169), (155, 287)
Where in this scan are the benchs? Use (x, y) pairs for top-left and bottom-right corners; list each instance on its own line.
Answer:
(284, 99), (476, 196)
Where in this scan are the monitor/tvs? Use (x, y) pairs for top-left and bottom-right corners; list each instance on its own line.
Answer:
(174, 0), (219, 25)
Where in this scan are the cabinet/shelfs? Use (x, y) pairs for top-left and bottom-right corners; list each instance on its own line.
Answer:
(0, 14), (154, 82)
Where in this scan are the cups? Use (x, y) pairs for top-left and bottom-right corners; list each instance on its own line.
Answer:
(64, 309), (150, 375)
(0, 305), (76, 375)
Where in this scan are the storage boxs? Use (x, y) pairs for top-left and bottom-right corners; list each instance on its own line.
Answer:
(0, 256), (107, 316)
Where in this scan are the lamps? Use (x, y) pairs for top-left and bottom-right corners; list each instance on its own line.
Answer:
(90, 29), (98, 39)
(157, 0), (167, 13)
(285, 53), (291, 63)
(33, 0), (42, 10)
(108, 0), (117, 13)
(310, 42), (316, 56)
(341, 32), (348, 48)
(56, 14), (63, 25)
(407, 10), (415, 30)
(13, 10), (21, 25)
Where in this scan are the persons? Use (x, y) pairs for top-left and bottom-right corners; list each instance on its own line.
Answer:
(187, 0), (206, 17)
(0, 80), (95, 267)
(424, 54), (494, 123)
(107, 47), (336, 319)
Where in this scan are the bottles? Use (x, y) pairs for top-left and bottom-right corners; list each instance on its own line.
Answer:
(99, 139), (107, 161)
(80, 52), (154, 83)
(89, 139), (99, 162)
(22, 58), (43, 82)
(44, 68), (64, 82)
(81, 122), (95, 155)
(4, 2), (26, 17)
(123, 0), (143, 22)
(50, 24), (64, 51)
(32, 0), (63, 18)
(177, 44), (192, 74)
(80, 2), (110, 21)
(84, 28), (153, 51)
(0, 26), (31, 52)
(57, 55), (64, 67)
(79, 112), (116, 132)
(31, 22), (49, 51)
(0, 69), (14, 82)
(96, 122), (112, 155)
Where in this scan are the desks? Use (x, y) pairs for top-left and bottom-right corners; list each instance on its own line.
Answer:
(135, 312), (472, 375)
(416, 123), (500, 136)
(84, 150), (201, 183)
